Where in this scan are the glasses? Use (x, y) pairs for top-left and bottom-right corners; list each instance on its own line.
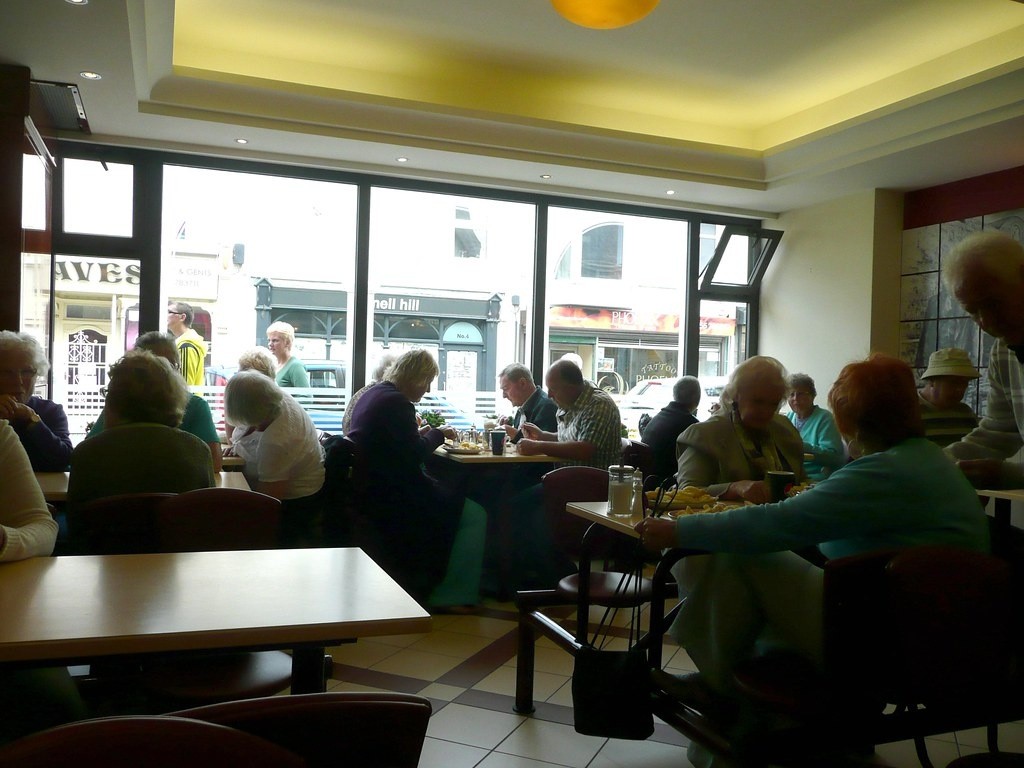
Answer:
(167, 310), (184, 314)
(0, 368), (37, 378)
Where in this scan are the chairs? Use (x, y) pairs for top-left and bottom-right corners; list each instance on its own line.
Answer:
(0, 430), (1024, 768)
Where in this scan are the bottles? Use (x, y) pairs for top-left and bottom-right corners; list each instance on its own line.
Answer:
(632, 467), (643, 515)
(607, 464), (635, 515)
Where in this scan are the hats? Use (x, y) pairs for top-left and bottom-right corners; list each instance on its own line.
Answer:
(920, 347), (983, 380)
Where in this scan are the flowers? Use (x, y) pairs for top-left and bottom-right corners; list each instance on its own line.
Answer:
(418, 409), (447, 429)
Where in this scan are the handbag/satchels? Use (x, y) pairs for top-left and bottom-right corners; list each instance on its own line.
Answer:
(571, 647), (656, 741)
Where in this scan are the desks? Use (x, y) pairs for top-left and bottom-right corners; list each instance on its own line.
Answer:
(974, 488), (1023, 525)
(432, 444), (558, 600)
(221, 443), (245, 466)
(35, 472), (250, 503)
(565, 499), (758, 678)
(0, 548), (432, 693)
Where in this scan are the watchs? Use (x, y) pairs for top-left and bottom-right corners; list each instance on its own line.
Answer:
(26, 413), (40, 426)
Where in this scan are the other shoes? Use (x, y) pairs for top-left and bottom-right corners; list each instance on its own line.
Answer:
(425, 602), (484, 615)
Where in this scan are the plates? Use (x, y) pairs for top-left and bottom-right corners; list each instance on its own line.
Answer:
(666, 511), (688, 520)
(645, 496), (720, 510)
(442, 446), (480, 454)
(790, 484), (826, 499)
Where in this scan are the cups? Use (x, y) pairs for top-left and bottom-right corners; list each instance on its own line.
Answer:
(490, 430), (505, 455)
(766, 470), (794, 505)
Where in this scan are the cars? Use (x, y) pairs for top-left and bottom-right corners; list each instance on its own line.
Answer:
(617, 375), (729, 441)
(202, 358), (486, 448)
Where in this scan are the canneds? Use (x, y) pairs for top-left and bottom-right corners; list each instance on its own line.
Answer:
(766, 470), (794, 502)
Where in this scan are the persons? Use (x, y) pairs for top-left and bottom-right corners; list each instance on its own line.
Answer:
(939, 230), (1024, 492)
(0, 330), (329, 563)
(342, 348), (482, 613)
(635, 346), (982, 768)
(265, 322), (314, 409)
(167, 302), (208, 394)
(485, 361), (621, 601)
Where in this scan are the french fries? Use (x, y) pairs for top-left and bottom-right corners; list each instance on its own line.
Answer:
(677, 500), (756, 515)
